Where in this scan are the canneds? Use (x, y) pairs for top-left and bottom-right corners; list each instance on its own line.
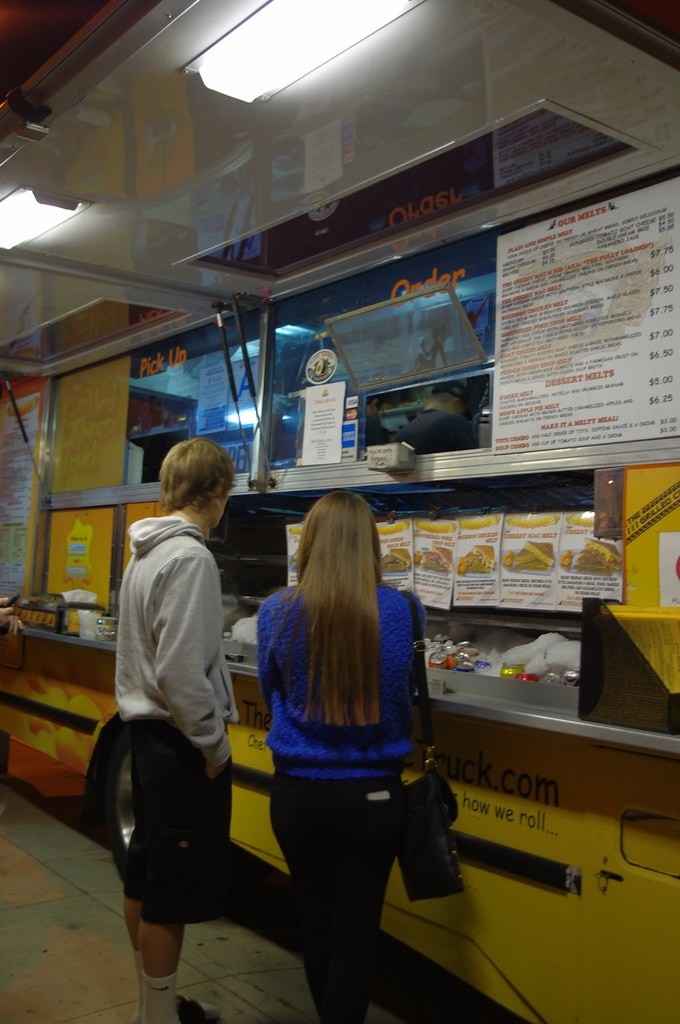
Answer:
(425, 634), (580, 688)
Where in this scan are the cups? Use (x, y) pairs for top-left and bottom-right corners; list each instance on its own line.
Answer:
(77, 610), (99, 640)
(96, 617), (118, 641)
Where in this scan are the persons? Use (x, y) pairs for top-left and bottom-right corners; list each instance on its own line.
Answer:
(254, 488), (424, 1023)
(113, 434), (240, 1022)
(364, 395), (391, 448)
(393, 381), (478, 455)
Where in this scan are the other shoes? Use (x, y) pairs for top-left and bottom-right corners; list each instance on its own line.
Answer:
(174, 994), (223, 1024)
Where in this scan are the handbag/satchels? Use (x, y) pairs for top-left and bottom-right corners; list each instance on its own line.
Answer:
(396, 769), (463, 901)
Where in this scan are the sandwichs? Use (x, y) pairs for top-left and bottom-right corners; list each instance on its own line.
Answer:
(382, 538), (622, 576)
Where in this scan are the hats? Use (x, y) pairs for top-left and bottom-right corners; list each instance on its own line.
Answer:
(433, 379), (473, 422)
